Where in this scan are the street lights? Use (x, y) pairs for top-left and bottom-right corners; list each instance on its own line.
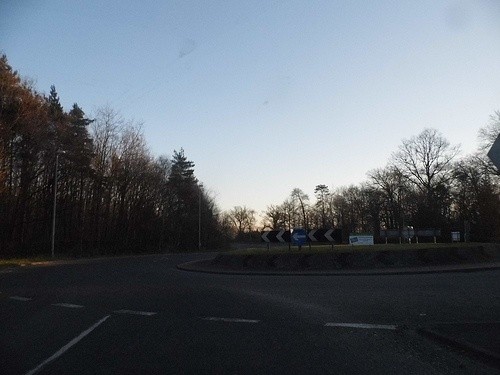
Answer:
(51, 151), (65, 258)
(198, 184), (203, 250)
